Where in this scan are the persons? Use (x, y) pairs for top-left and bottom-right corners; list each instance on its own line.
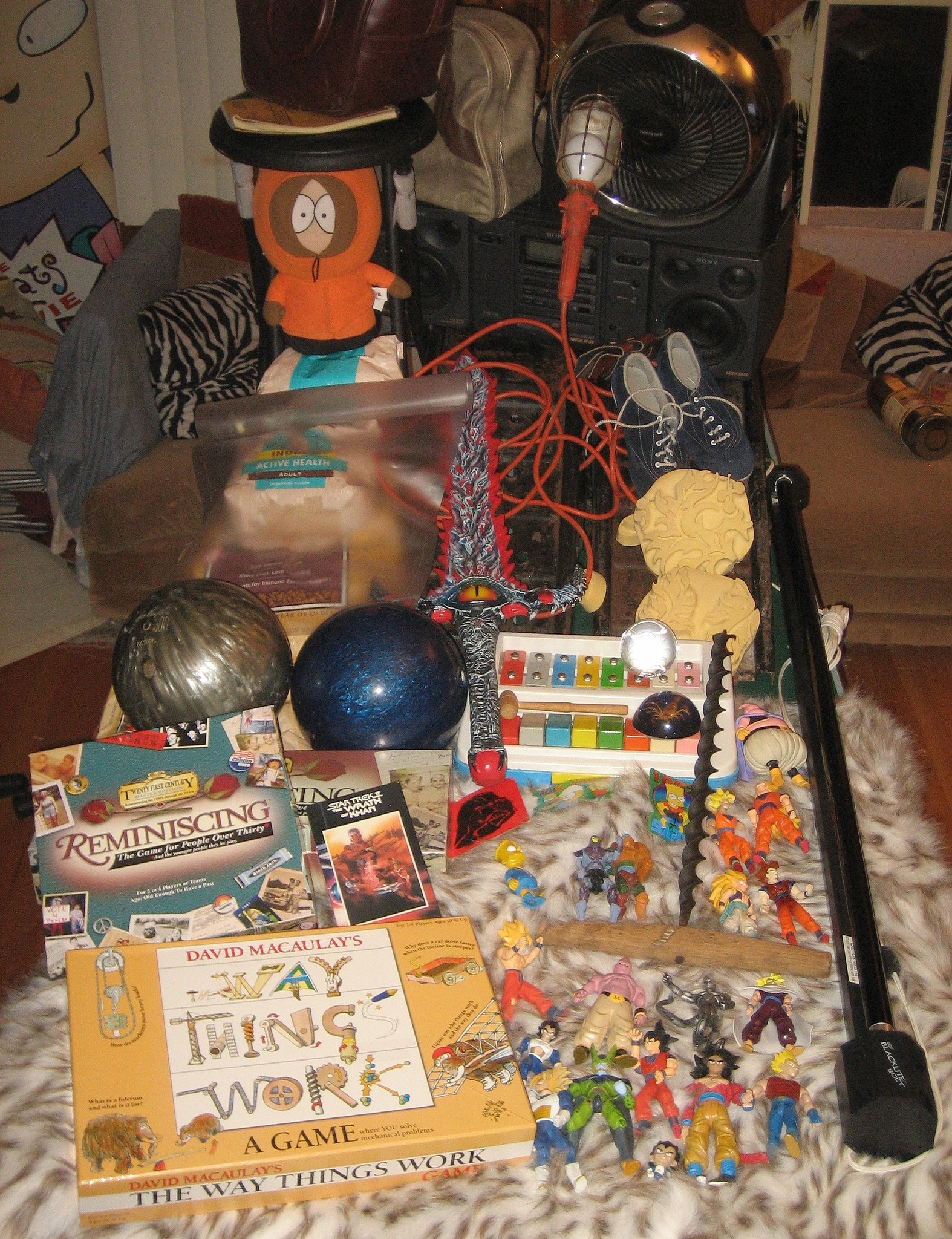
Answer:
(496, 840), (546, 911)
(738, 973), (806, 1056)
(630, 1019), (683, 1142)
(527, 1064), (588, 1195)
(678, 1037), (755, 1188)
(573, 957), (646, 1069)
(657, 973), (737, 1049)
(739, 1051), (822, 1163)
(497, 919), (569, 1030)
(512, 1019), (562, 1090)
(564, 1043), (642, 1181)
(646, 1140), (682, 1181)
(571, 695), (833, 947)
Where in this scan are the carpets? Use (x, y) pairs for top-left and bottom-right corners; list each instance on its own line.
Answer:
(0, 689), (951, 1239)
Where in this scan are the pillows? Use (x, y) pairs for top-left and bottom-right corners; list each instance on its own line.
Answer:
(0, 0), (123, 337)
(853, 255), (952, 389)
(177, 190), (251, 291)
(136, 271), (258, 440)
(0, 276), (109, 675)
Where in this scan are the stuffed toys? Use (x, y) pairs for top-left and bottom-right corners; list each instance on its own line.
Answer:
(250, 164), (414, 356)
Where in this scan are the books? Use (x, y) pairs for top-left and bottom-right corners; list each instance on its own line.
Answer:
(21, 704), (456, 985)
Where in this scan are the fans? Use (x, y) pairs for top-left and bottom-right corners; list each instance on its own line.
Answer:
(531, 0), (796, 252)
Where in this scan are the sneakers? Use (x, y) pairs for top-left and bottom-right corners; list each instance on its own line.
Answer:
(657, 330), (754, 483)
(611, 351), (689, 498)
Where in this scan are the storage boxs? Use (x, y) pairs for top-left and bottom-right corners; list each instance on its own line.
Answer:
(423, 325), (583, 635)
(63, 917), (538, 1224)
(587, 351), (771, 680)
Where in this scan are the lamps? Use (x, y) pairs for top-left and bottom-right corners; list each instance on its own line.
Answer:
(553, 93), (620, 301)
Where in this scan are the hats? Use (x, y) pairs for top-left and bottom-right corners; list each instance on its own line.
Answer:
(55, 898), (63, 901)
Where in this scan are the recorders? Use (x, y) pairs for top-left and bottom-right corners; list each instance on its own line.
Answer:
(372, 114), (795, 383)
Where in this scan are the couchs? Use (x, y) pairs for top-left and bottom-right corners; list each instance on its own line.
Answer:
(31, 208), (952, 649)
